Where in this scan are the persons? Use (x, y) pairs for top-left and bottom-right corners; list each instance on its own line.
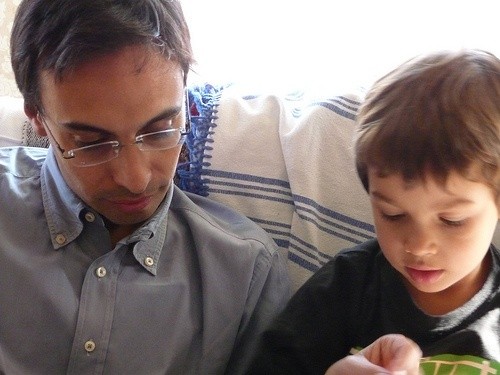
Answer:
(0, 2), (289, 375)
(248, 49), (500, 375)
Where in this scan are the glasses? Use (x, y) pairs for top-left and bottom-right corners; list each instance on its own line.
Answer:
(29, 73), (192, 167)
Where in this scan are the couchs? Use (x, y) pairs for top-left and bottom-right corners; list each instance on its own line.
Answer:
(0, 84), (500, 302)
